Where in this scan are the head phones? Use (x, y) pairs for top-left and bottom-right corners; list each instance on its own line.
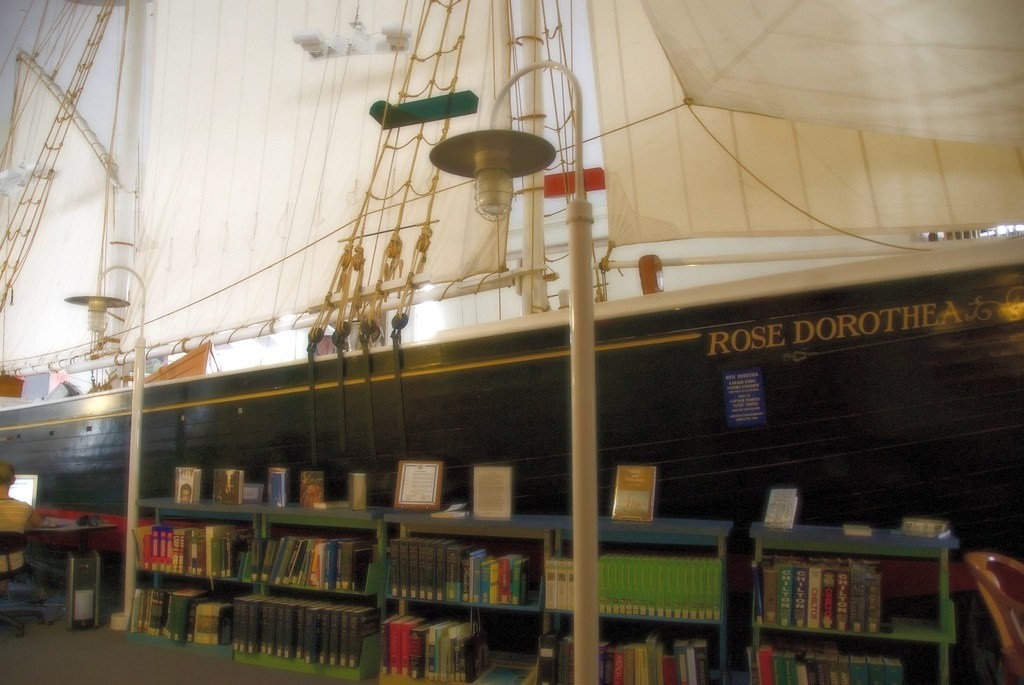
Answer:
(76, 514), (104, 526)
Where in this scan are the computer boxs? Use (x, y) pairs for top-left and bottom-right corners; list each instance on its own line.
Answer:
(65, 548), (100, 629)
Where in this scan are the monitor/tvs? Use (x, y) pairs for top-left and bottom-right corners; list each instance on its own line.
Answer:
(8, 475), (38, 511)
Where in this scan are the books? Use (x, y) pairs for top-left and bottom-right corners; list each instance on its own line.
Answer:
(749, 554), (883, 634)
(610, 465), (656, 522)
(900, 517), (952, 538)
(297, 471), (367, 511)
(764, 488), (799, 531)
(387, 537), (535, 605)
(431, 502), (469, 519)
(844, 524), (873, 536)
(747, 645), (903, 685)
(472, 466), (512, 521)
(229, 593), (378, 668)
(174, 467), (202, 503)
(378, 616), (483, 683)
(266, 468), (289, 507)
(130, 586), (231, 646)
(212, 469), (244, 506)
(543, 549), (724, 622)
(131, 522), (370, 590)
(535, 633), (711, 685)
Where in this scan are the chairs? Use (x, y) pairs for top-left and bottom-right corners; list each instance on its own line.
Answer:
(0, 530), (46, 638)
(962, 551), (1024, 685)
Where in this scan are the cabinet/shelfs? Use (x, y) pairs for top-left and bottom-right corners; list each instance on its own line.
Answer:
(233, 505), (401, 680)
(377, 513), (571, 685)
(748, 522), (960, 685)
(553, 516), (734, 685)
(124, 496), (266, 662)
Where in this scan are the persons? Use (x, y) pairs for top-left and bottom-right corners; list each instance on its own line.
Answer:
(0, 459), (68, 620)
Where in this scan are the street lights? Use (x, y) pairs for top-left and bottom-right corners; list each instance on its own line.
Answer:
(429, 61), (602, 685)
(64, 264), (146, 632)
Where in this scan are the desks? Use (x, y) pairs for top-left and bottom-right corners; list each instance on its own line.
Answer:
(24, 516), (118, 625)
